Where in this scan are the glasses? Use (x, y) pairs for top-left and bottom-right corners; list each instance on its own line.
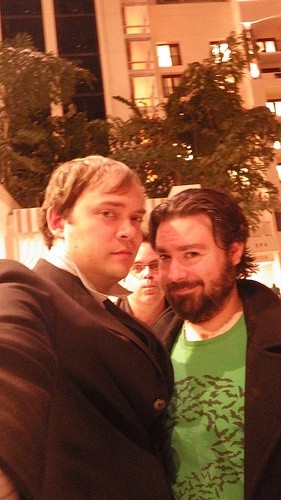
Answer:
(130, 261), (164, 274)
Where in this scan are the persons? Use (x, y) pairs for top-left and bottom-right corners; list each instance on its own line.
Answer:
(116, 232), (184, 327)
(148, 188), (281, 500)
(0, 155), (175, 500)
